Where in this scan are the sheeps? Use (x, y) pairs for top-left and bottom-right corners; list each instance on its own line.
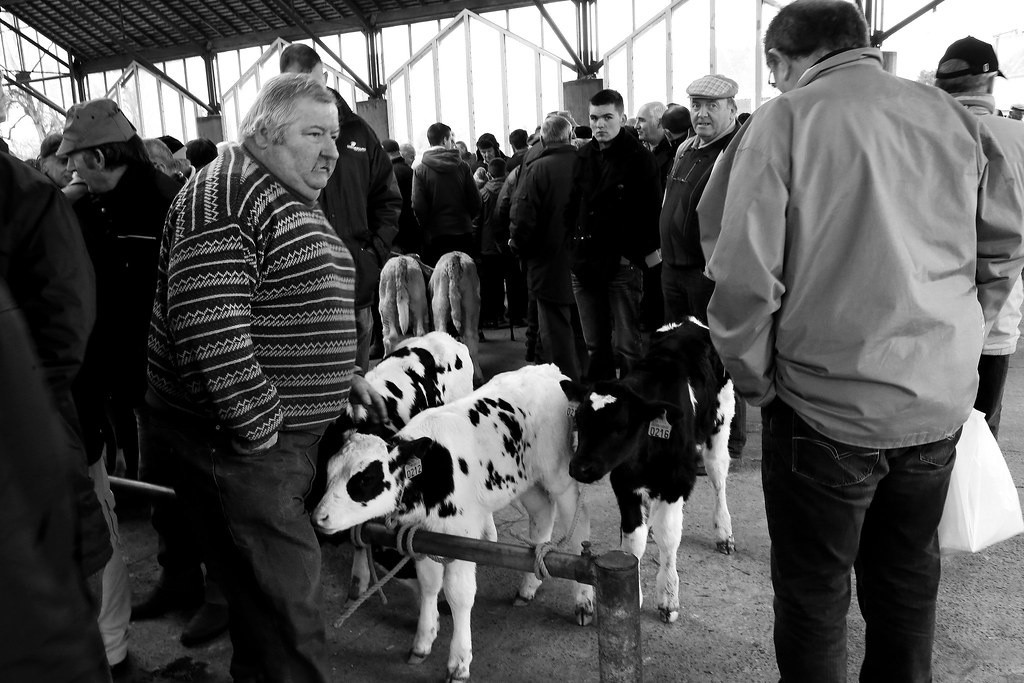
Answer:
(559, 316), (736, 622)
(311, 363), (594, 683)
(343, 331), (474, 609)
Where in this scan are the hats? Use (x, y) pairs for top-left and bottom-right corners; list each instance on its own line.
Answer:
(686, 74), (739, 99)
(55, 97), (137, 157)
(935, 35), (1008, 80)
(382, 140), (399, 152)
(476, 133), (501, 161)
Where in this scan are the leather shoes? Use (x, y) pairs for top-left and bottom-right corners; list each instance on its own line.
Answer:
(178, 598), (230, 648)
(130, 571), (179, 620)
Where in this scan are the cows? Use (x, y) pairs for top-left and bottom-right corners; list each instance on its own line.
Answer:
(378, 251), (486, 384)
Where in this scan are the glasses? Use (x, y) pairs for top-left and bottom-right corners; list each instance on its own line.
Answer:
(768, 70), (776, 88)
(667, 148), (701, 183)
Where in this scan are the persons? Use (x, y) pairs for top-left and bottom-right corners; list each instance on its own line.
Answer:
(146, 72), (358, 682)
(564, 88), (664, 390)
(0, 0), (1024, 683)
